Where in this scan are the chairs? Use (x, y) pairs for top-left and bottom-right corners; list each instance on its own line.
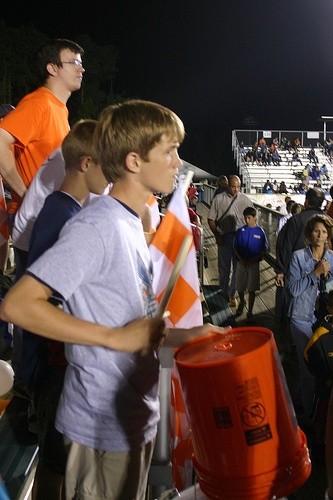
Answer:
(187, 187), (204, 288)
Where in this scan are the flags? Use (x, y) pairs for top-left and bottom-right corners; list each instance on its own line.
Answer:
(143, 187), (197, 494)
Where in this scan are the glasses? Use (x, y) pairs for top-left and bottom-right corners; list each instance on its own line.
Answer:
(61, 59), (82, 67)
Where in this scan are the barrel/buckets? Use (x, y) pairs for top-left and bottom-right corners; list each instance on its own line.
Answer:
(173, 329), (311, 500)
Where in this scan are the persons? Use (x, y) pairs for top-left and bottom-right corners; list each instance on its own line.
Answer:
(233, 207), (270, 325)
(280, 187), (333, 349)
(9, 147), (66, 446)
(138, 175), (210, 268)
(213, 176), (228, 199)
(0, 38), (86, 403)
(11, 119), (110, 500)
(236, 132), (333, 235)
(303, 289), (333, 467)
(0, 100), (232, 500)
(280, 214), (333, 431)
(208, 175), (257, 301)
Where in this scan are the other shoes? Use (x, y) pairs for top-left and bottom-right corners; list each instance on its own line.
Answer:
(235, 300), (247, 315)
(246, 311), (254, 322)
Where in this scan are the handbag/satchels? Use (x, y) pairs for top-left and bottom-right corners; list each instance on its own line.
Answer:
(275, 280), (288, 310)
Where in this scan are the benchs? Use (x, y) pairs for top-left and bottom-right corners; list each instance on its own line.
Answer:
(232, 145), (333, 194)
